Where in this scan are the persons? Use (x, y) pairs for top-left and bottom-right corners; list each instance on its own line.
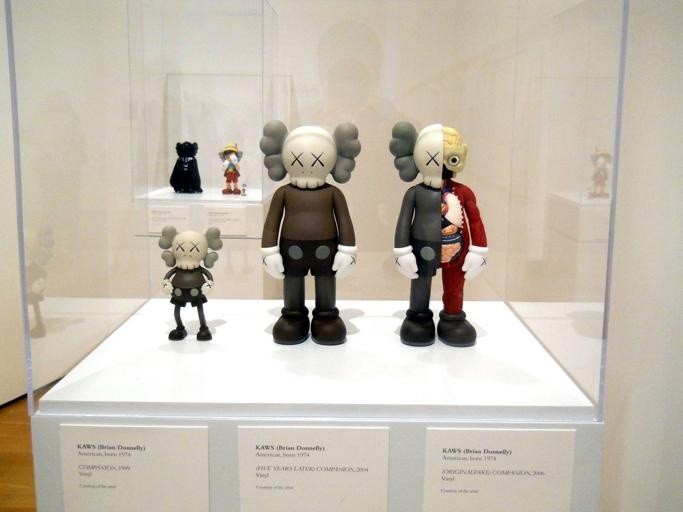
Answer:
(257, 118), (361, 346)
(23, 224), (54, 338)
(387, 120), (488, 349)
(156, 224), (224, 342)
(219, 141), (244, 195)
(586, 144), (615, 201)
